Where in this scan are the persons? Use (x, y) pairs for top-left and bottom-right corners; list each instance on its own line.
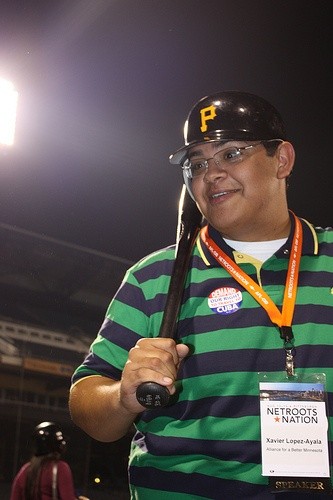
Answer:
(68, 90), (332, 499)
(10, 420), (92, 500)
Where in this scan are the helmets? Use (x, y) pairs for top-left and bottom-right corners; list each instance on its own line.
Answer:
(27, 422), (70, 457)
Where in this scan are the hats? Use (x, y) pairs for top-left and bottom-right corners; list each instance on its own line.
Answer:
(169, 91), (291, 166)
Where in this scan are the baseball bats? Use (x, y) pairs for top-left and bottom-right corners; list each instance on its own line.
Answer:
(136, 183), (203, 408)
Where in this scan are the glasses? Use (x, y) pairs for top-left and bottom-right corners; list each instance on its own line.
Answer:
(183, 139), (284, 178)
